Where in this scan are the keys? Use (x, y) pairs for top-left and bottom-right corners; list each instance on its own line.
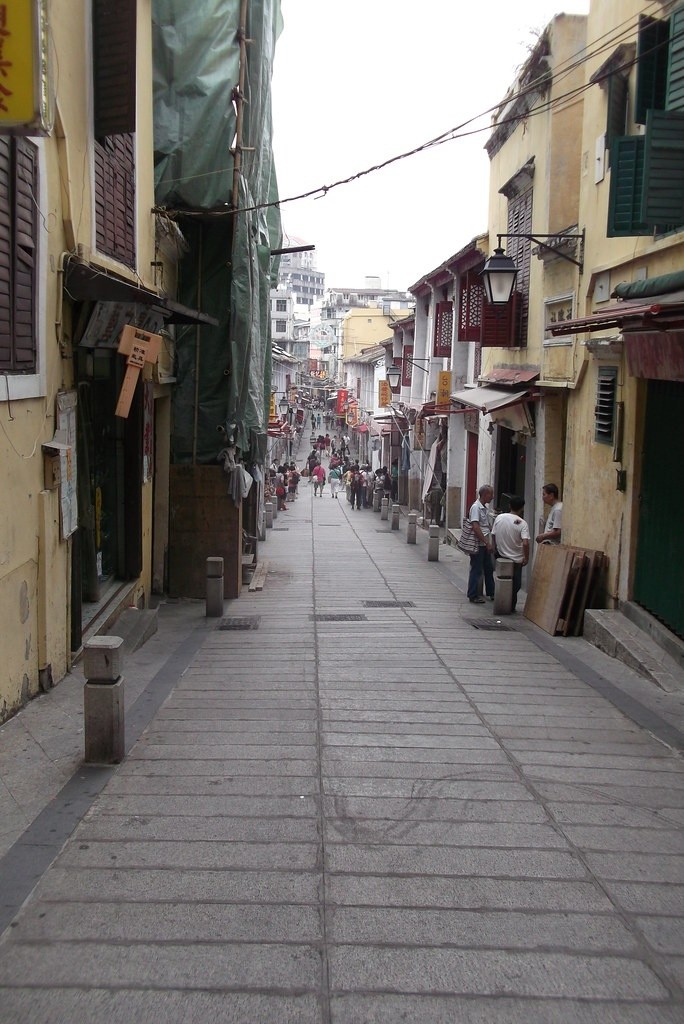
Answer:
(115, 324), (163, 418)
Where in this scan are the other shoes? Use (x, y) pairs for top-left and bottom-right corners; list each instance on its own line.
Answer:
(491, 593), (494, 600)
(469, 597), (486, 603)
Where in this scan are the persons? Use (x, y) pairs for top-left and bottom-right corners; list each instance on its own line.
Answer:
(491, 496), (530, 612)
(269, 388), (400, 512)
(536, 483), (563, 544)
(465, 485), (494, 603)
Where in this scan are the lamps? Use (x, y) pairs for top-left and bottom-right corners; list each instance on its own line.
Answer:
(271, 392), (298, 415)
(478, 233), (584, 305)
(343, 399), (348, 410)
(387, 358), (429, 387)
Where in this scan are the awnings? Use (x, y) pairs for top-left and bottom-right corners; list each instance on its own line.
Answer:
(448, 383), (530, 414)
(368, 400), (449, 426)
(65, 259), (220, 326)
(355, 424), (368, 433)
(543, 295), (683, 336)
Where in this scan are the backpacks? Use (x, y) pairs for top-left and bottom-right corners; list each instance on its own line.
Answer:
(290, 472), (300, 484)
(351, 472), (361, 487)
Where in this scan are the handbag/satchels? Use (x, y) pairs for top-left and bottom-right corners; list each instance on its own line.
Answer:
(458, 504), (480, 554)
(311, 474), (318, 483)
